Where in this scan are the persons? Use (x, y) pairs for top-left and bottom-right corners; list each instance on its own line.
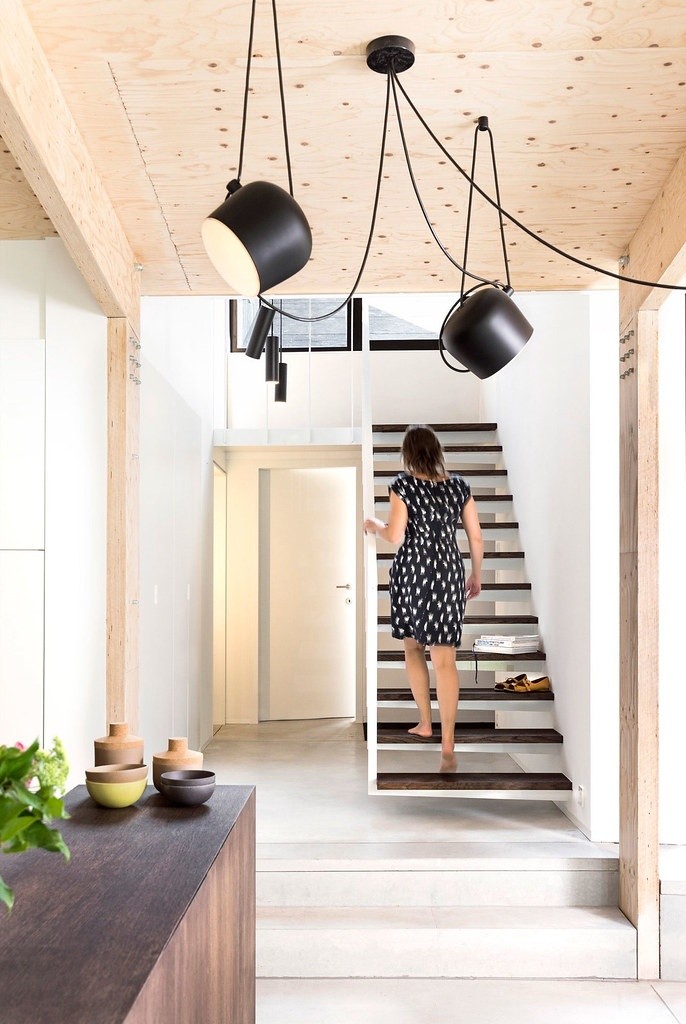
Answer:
(364, 427), (484, 773)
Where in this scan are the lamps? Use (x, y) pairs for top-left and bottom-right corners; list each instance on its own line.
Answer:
(242, 304), (289, 405)
(439, 115), (534, 380)
(203, 0), (312, 296)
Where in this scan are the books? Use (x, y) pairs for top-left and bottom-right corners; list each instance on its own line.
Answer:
(473, 635), (541, 654)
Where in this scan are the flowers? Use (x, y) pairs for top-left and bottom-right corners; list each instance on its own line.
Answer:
(0, 738), (72, 918)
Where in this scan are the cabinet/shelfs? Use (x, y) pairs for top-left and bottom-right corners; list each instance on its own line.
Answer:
(0, 786), (256, 1024)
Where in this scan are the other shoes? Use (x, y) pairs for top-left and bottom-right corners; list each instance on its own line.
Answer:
(504, 674), (550, 692)
(493, 674), (527, 691)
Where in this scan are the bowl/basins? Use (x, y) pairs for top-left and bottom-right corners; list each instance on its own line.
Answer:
(84, 763), (148, 808)
(159, 771), (215, 807)
